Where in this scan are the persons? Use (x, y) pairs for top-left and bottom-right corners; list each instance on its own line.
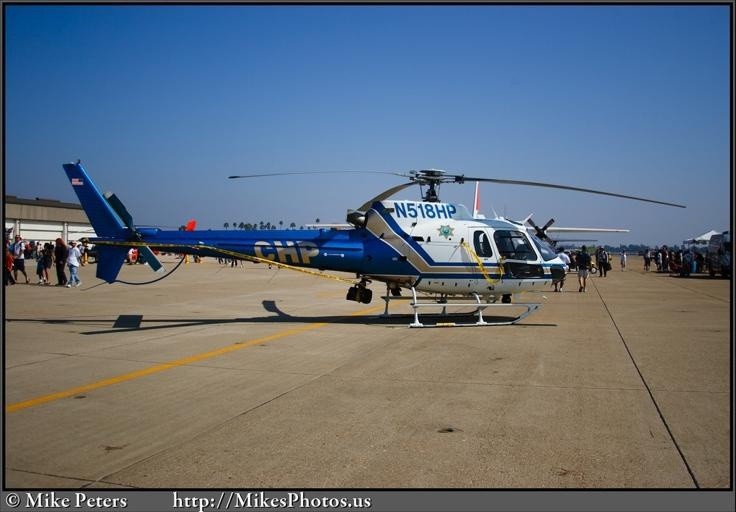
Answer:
(620, 250), (626, 271)
(550, 244), (612, 292)
(644, 245), (704, 273)
(4, 227), (245, 288)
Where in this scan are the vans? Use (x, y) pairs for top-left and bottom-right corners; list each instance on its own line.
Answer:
(707, 229), (731, 279)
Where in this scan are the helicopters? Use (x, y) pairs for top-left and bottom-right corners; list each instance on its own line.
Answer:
(62, 160), (688, 328)
